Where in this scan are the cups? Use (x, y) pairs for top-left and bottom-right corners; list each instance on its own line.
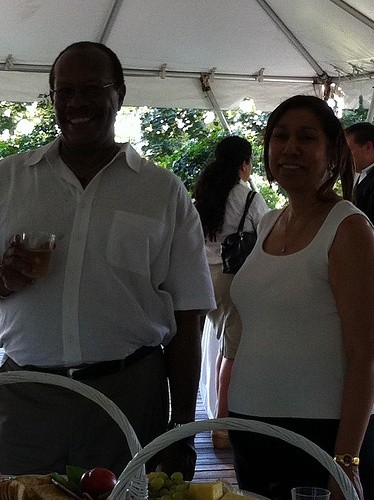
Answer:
(21, 231), (56, 279)
(291, 487), (331, 500)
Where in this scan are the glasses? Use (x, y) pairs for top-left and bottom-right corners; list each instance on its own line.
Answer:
(48, 82), (115, 99)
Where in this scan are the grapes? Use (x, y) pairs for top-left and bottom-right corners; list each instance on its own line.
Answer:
(142, 471), (189, 500)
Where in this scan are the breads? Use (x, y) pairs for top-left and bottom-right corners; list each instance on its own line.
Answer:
(0, 475), (80, 500)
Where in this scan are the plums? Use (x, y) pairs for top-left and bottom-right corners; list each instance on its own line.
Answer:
(79, 468), (117, 500)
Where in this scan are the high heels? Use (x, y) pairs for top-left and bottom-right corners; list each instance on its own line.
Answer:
(211, 435), (231, 449)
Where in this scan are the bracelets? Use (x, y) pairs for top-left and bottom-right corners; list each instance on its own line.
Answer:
(0, 296), (7, 302)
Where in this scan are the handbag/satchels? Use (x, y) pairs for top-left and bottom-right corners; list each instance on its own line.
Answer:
(220, 191), (257, 274)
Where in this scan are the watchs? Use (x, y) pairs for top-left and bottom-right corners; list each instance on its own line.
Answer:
(335, 454), (360, 467)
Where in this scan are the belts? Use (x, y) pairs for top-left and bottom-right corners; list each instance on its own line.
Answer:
(24, 347), (154, 382)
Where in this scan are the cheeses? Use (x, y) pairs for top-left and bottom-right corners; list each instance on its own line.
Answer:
(185, 482), (257, 500)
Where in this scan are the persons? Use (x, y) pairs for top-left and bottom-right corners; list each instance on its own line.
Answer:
(192, 136), (271, 449)
(229, 95), (374, 500)
(0, 41), (217, 480)
(345, 122), (374, 225)
